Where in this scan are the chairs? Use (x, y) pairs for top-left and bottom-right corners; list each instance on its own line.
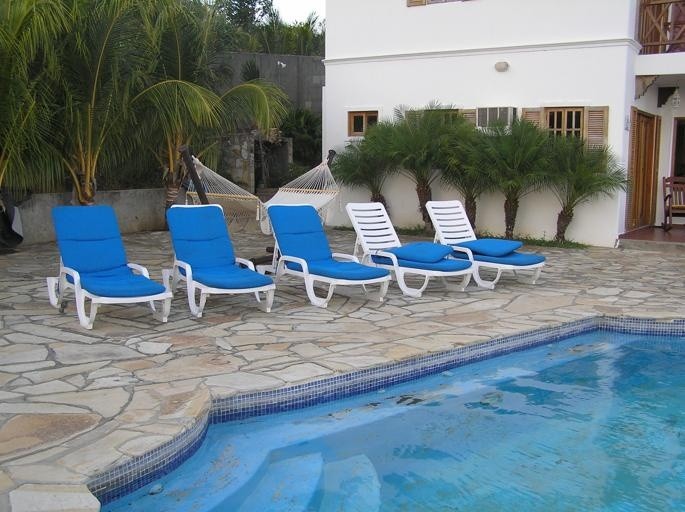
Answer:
(661, 176), (685, 232)
(45, 204), (174, 330)
(344, 203), (476, 299)
(255, 204), (392, 308)
(425, 201), (545, 291)
(162, 204), (277, 318)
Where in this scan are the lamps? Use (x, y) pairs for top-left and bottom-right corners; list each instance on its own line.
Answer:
(671, 83), (681, 114)
